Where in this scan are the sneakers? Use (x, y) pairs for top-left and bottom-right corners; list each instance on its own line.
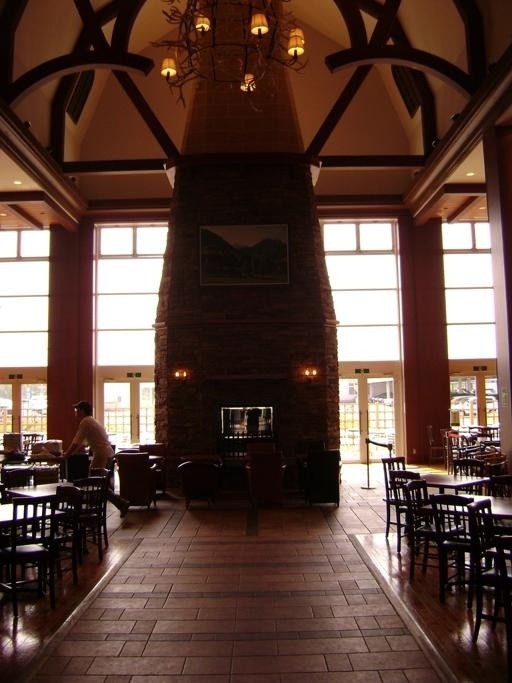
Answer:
(120, 499), (130, 518)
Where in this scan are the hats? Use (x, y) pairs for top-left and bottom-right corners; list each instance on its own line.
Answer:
(71, 401), (91, 408)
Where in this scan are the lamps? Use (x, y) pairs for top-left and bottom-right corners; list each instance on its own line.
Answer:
(148, 0), (310, 107)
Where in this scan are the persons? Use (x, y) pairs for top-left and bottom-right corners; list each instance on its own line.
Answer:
(61, 400), (131, 518)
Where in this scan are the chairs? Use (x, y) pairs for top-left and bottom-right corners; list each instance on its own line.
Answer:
(383, 427), (512, 683)
(0, 433), (115, 618)
(113, 441), (339, 510)
